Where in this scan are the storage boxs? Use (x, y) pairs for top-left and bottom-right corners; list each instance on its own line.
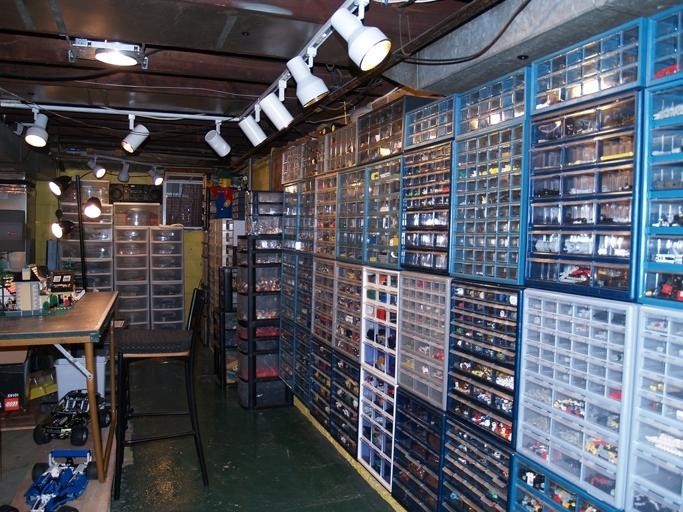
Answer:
(52, 355), (108, 403)
(58, 177), (184, 330)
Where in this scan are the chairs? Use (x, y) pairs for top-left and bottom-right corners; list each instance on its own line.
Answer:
(113, 284), (208, 497)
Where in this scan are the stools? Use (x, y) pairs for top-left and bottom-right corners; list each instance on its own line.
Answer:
(0, 347), (32, 415)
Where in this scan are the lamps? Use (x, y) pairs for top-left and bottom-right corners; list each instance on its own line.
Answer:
(47, 176), (104, 292)
(65, 33), (150, 72)
(236, 1), (394, 149)
(85, 154), (166, 189)
(1, 97), (243, 160)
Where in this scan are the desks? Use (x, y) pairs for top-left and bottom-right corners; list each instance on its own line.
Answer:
(0, 290), (120, 482)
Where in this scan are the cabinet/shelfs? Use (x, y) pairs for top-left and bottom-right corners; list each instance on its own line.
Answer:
(200, 192), (297, 408)
(390, 0), (681, 512)
(278, 98), (404, 495)
